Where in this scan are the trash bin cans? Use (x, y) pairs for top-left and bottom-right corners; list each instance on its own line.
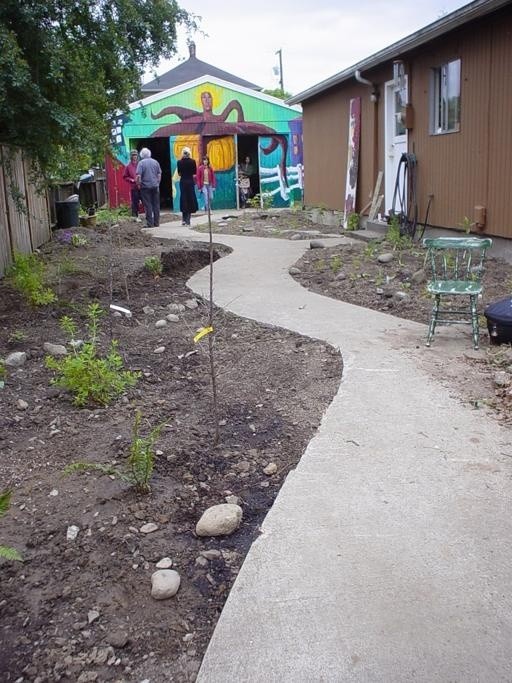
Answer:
(55, 202), (80, 229)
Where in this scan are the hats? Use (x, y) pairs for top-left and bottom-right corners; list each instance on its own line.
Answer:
(130, 149), (138, 160)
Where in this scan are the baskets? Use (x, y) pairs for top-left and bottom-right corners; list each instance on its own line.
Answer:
(80, 215), (97, 228)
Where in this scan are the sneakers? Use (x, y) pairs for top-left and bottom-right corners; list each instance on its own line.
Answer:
(205, 210), (212, 215)
(181, 221), (190, 227)
(143, 224), (159, 228)
(131, 217), (142, 223)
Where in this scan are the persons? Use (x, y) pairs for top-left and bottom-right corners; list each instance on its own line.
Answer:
(238, 155), (255, 208)
(122, 149), (144, 223)
(134, 147), (162, 228)
(196, 155), (217, 215)
(177, 146), (198, 227)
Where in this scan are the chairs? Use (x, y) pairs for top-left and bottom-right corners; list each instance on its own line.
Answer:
(421, 236), (492, 349)
